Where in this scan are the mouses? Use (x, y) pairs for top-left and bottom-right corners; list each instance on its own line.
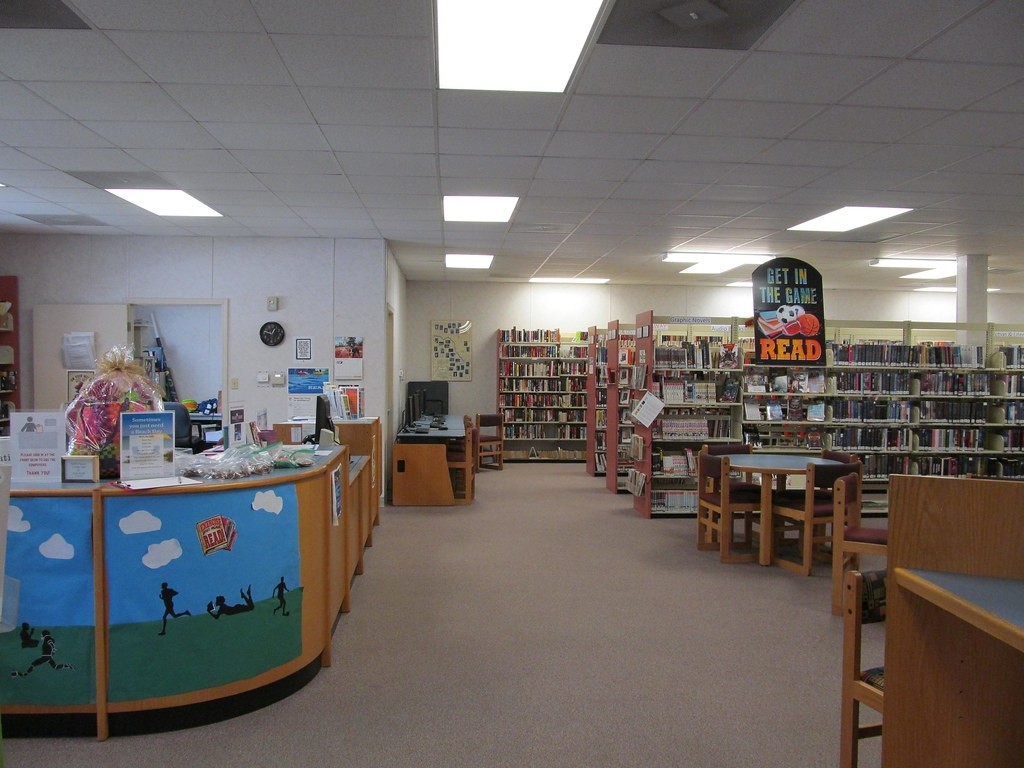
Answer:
(439, 427), (448, 430)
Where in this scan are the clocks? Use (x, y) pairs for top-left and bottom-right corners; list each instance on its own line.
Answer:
(259, 321), (285, 347)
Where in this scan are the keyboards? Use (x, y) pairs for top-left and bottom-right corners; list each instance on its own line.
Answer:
(415, 427), (429, 434)
(430, 416), (445, 427)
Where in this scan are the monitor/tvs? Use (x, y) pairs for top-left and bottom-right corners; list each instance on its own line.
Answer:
(404, 389), (425, 433)
(314, 394), (336, 444)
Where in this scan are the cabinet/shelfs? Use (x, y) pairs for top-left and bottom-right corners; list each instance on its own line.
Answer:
(0, 275), (21, 436)
(496, 310), (1024, 519)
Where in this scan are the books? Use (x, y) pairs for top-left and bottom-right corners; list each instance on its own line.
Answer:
(832, 342), (997, 479)
(651, 478), (698, 511)
(652, 447), (696, 477)
(729, 470), (792, 488)
(739, 338), (826, 449)
(651, 408), (731, 440)
(654, 335), (739, 369)
(324, 382), (365, 420)
(500, 326), (588, 439)
(1000, 345), (1024, 481)
(620, 339), (647, 497)
(653, 370), (738, 403)
(595, 334), (607, 472)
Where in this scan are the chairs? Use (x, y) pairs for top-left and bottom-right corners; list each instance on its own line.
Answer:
(474, 413), (504, 473)
(771, 460), (861, 578)
(696, 451), (778, 563)
(773, 449), (857, 551)
(447, 415), (476, 505)
(702, 444), (777, 547)
(832, 473), (885, 616)
(840, 569), (886, 768)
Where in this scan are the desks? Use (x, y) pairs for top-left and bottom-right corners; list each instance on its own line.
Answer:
(393, 414), (466, 507)
(882, 474), (1024, 768)
(706, 454), (845, 566)
